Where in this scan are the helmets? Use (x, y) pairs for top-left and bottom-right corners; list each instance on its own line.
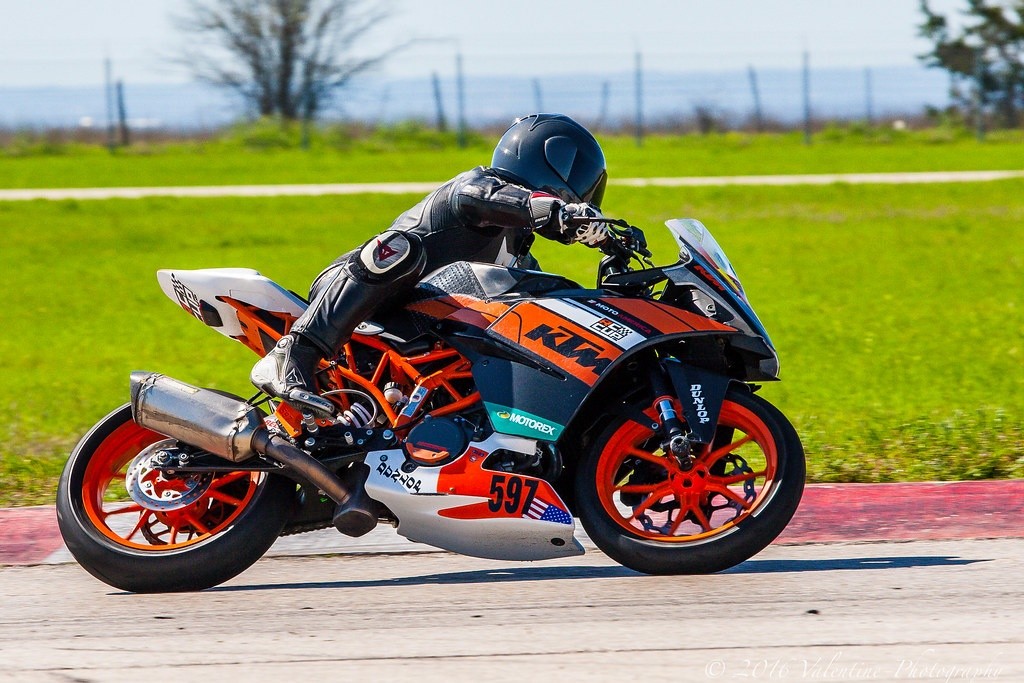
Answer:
(490, 112), (607, 246)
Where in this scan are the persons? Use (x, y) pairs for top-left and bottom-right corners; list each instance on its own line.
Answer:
(250, 114), (608, 416)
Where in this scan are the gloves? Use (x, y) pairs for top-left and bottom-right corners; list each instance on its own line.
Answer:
(559, 202), (608, 248)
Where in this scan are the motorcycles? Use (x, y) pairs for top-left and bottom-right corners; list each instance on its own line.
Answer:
(53, 210), (806, 592)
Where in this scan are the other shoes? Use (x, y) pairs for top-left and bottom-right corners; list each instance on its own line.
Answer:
(249, 335), (334, 419)
